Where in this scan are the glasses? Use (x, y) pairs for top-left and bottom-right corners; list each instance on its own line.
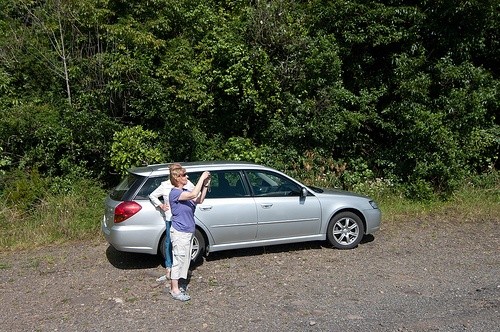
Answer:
(178, 173), (187, 177)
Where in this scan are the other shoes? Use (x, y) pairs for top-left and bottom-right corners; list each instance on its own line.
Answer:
(170, 289), (191, 301)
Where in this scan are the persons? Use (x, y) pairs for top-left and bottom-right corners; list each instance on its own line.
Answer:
(169, 166), (212, 301)
(148, 163), (201, 280)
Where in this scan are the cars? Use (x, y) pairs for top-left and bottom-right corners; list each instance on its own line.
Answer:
(102, 162), (384, 265)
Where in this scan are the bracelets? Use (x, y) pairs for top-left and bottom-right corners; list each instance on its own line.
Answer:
(204, 184), (208, 187)
